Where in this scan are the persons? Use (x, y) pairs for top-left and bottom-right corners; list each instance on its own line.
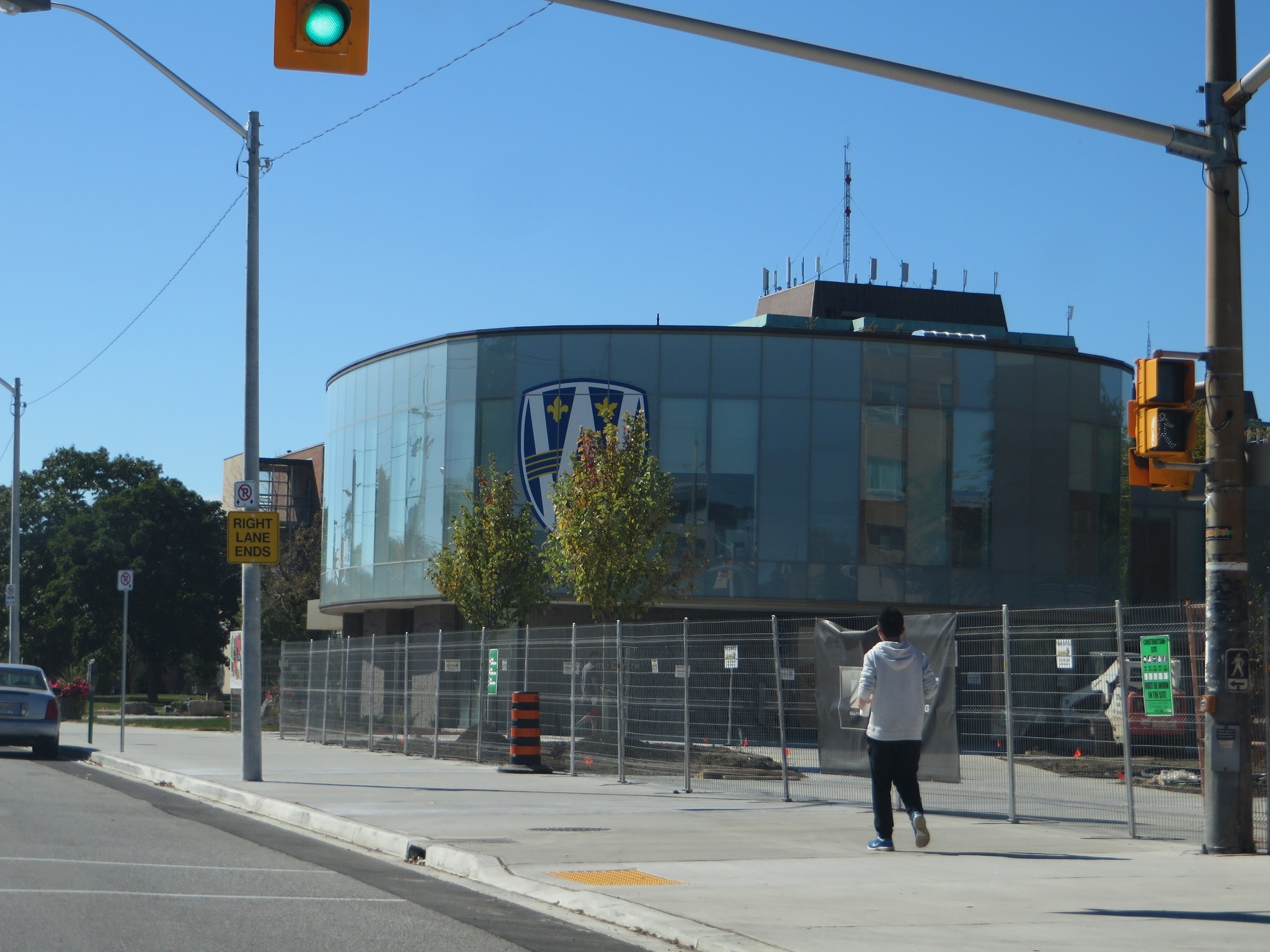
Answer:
(858, 605), (940, 852)
(574, 648), (602, 736)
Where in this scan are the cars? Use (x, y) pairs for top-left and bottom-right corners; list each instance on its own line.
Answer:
(0, 662), (60, 760)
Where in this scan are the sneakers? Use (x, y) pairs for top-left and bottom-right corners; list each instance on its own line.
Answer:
(910, 811), (930, 848)
(867, 832), (895, 851)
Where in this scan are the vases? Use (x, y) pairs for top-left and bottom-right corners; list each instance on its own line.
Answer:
(58, 698), (87, 721)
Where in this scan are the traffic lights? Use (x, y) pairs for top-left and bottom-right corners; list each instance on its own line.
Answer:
(1136, 355), (1196, 452)
(274, 0), (371, 76)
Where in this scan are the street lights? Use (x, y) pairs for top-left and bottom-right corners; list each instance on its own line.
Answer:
(1, 0), (266, 783)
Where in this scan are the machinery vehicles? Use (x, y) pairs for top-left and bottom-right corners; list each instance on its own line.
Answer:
(1046, 651), (1189, 754)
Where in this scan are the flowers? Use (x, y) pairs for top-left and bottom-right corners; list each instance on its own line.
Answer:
(48, 664), (91, 699)
(266, 674), (298, 699)
